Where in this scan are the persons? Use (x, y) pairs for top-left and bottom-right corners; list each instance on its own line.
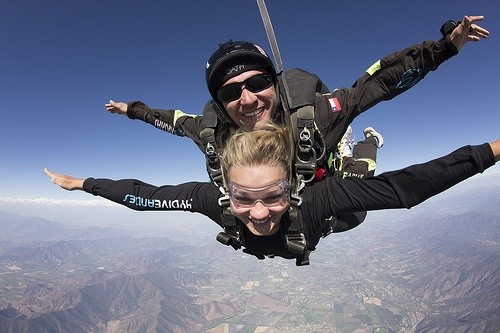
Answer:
(41, 116), (499, 267)
(104, 13), (491, 191)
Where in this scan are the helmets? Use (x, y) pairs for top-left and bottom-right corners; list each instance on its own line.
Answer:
(206, 40), (278, 118)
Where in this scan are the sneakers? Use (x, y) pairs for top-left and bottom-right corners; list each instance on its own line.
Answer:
(339, 126), (352, 143)
(364, 127), (384, 148)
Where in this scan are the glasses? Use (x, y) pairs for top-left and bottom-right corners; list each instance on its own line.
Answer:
(226, 178), (289, 207)
(216, 73), (274, 103)
